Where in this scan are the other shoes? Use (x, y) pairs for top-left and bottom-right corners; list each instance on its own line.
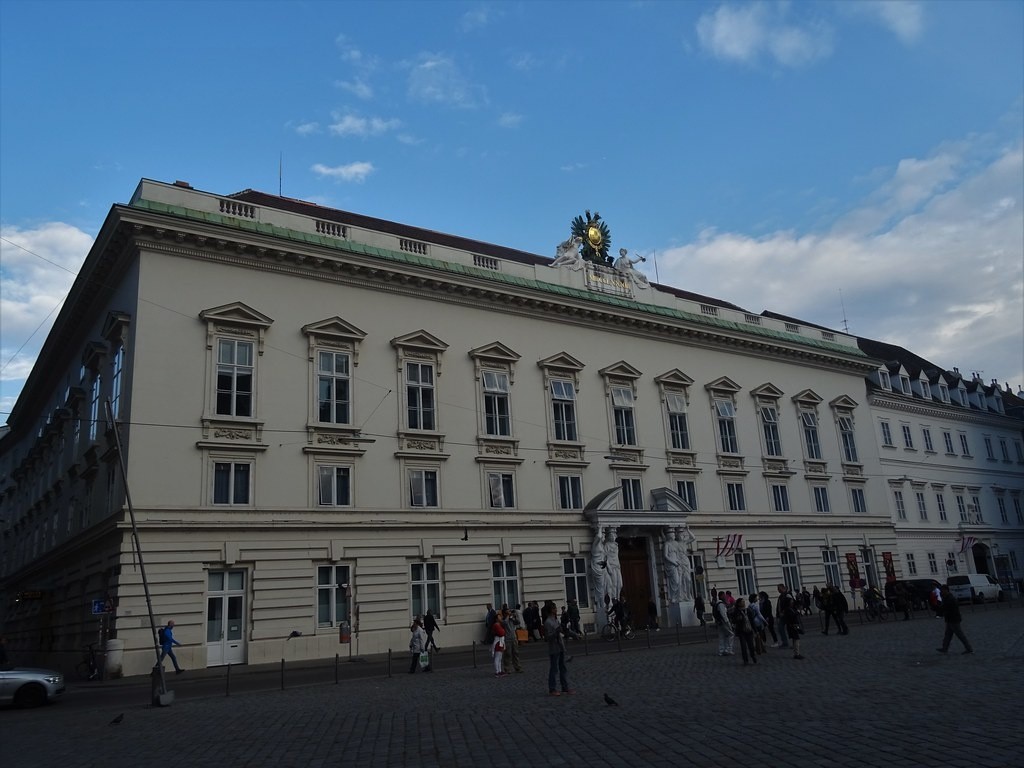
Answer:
(516, 669), (525, 673)
(779, 643), (789, 648)
(822, 630), (828, 636)
(838, 630), (849, 635)
(423, 668), (430, 672)
(562, 690), (576, 695)
(436, 647), (440, 652)
(549, 691), (560, 696)
(408, 670), (415, 673)
(753, 657), (757, 663)
(936, 648), (948, 653)
(771, 642), (779, 647)
(719, 652), (725, 656)
(725, 650), (735, 655)
(496, 672), (507, 677)
(794, 654), (806, 659)
(961, 651), (974, 655)
(176, 669), (184, 674)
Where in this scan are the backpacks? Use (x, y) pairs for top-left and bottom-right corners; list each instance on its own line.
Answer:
(487, 624), (497, 644)
(158, 628), (169, 644)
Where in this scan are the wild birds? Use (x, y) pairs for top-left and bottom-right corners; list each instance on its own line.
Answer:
(604, 693), (618, 706)
(287, 631), (302, 641)
(110, 713), (124, 724)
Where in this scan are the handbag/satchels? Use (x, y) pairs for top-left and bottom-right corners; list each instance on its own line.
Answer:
(419, 650), (429, 668)
(790, 624), (800, 633)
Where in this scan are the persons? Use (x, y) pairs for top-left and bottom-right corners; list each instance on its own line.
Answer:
(893, 582), (912, 621)
(663, 523), (695, 604)
(644, 598), (662, 631)
(693, 591), (706, 626)
(149, 620), (185, 675)
(547, 237), (583, 270)
(480, 600), (584, 645)
(492, 608), (525, 676)
(544, 602), (582, 696)
(778, 583), (806, 660)
(935, 585), (974, 655)
(424, 608), (441, 653)
(862, 585), (885, 609)
(605, 592), (638, 641)
(0, 636), (11, 665)
(813, 585), (849, 635)
(713, 590), (779, 666)
(905, 580), (928, 610)
(614, 248), (655, 290)
(794, 586), (811, 616)
(407, 619), (430, 674)
(590, 521), (623, 608)
(930, 583), (944, 619)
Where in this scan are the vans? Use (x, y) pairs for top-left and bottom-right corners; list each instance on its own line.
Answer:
(884, 579), (943, 611)
(945, 574), (1007, 605)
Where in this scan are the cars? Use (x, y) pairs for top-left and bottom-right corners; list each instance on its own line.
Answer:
(0, 664), (67, 710)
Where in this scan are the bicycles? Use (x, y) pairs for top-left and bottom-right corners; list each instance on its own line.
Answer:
(74, 641), (101, 681)
(865, 597), (889, 622)
(600, 614), (637, 642)
